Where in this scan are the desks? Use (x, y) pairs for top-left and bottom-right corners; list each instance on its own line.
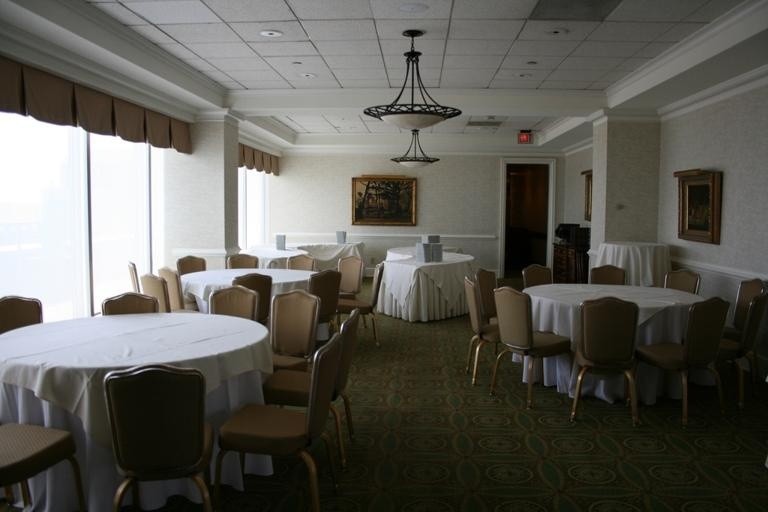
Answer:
(520, 283), (708, 408)
(0, 313), (272, 511)
(585, 241), (675, 291)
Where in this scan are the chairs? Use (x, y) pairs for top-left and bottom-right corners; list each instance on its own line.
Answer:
(126, 229), (388, 348)
(591, 264), (627, 287)
(102, 361), (214, 512)
(0, 293), (45, 336)
(572, 295), (642, 429)
(462, 265), (502, 386)
(267, 288), (320, 373)
(663, 268), (702, 296)
(493, 285), (571, 412)
(100, 291), (159, 317)
(717, 277), (766, 408)
(520, 263), (552, 287)
(216, 333), (345, 512)
(278, 307), (360, 442)
(626, 296), (731, 431)
(0, 420), (86, 512)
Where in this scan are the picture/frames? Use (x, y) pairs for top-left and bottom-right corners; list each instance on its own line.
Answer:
(350, 174), (418, 229)
(672, 167), (724, 249)
(580, 169), (593, 220)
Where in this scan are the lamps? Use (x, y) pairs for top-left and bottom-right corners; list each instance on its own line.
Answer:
(388, 126), (439, 169)
(359, 29), (465, 129)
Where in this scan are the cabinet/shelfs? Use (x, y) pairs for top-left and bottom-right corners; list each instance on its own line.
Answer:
(550, 241), (592, 286)
